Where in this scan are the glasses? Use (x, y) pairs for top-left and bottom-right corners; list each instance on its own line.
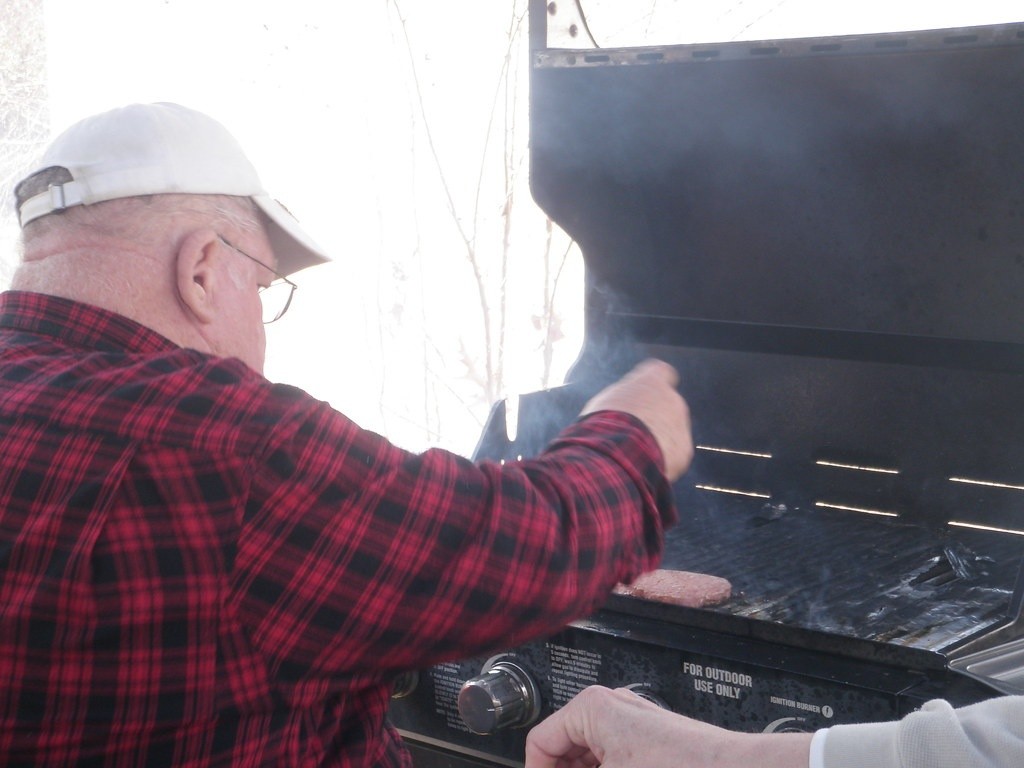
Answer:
(216, 233), (298, 324)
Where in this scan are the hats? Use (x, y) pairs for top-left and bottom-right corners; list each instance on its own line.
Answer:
(14, 100), (334, 283)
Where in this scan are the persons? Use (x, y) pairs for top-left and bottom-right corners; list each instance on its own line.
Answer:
(524, 684), (1024, 768)
(0, 103), (697, 768)
